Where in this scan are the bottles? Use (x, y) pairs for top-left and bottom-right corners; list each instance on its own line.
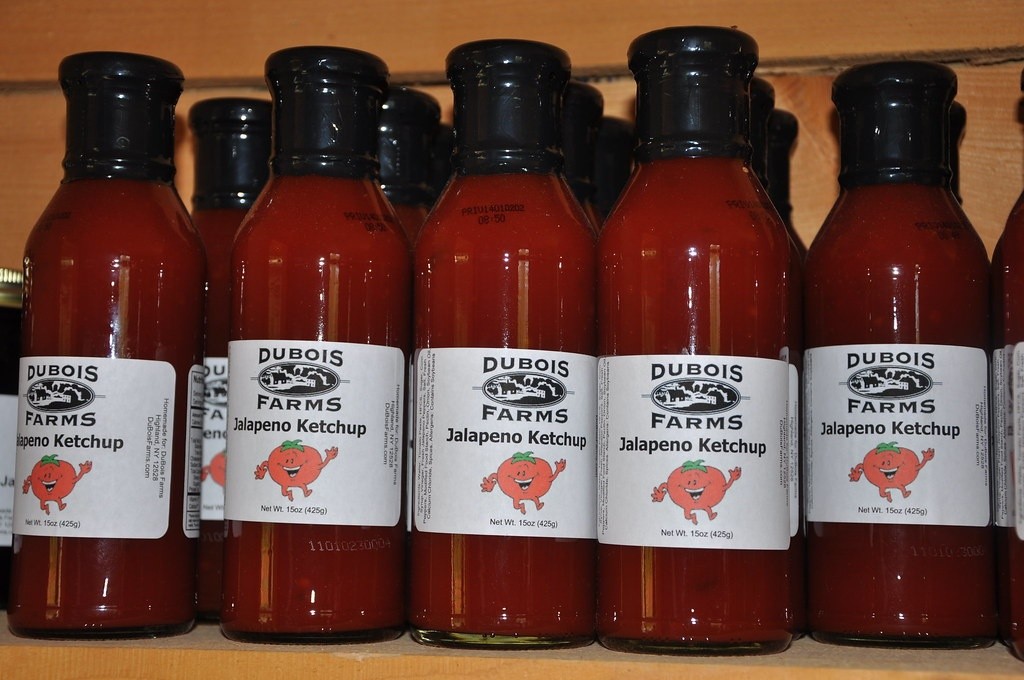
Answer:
(378, 24), (1024, 663)
(8, 51), (208, 640)
(214, 45), (414, 646)
(188, 95), (273, 627)
